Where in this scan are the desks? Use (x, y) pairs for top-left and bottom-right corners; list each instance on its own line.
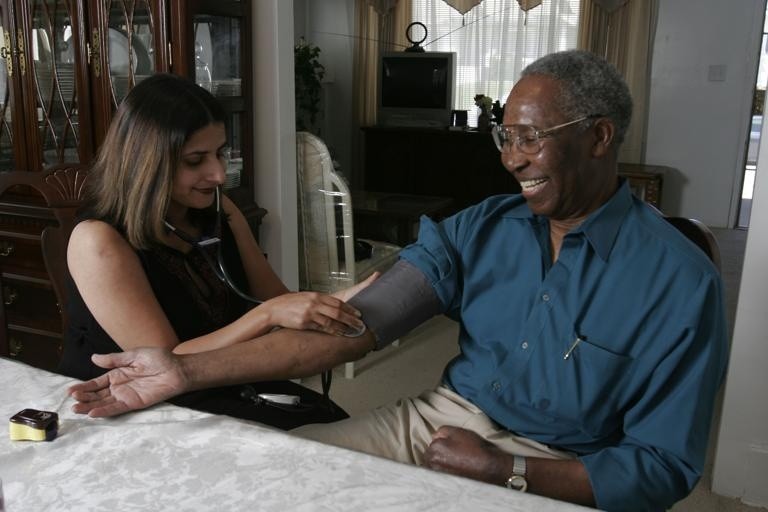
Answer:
(1, 358), (606, 512)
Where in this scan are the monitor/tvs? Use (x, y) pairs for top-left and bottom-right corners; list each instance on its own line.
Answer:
(376, 51), (452, 128)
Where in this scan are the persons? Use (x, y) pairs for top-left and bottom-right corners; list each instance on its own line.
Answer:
(63, 79), (365, 430)
(69, 49), (730, 512)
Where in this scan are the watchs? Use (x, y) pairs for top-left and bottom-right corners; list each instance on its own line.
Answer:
(503, 452), (530, 494)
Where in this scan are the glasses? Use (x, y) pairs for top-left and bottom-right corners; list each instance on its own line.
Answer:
(491, 115), (588, 155)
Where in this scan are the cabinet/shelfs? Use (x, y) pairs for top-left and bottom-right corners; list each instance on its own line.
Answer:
(1, 1), (254, 369)
(357, 119), (523, 204)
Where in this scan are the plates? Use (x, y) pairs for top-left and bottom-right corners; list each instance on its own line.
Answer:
(33, 24), (244, 191)
